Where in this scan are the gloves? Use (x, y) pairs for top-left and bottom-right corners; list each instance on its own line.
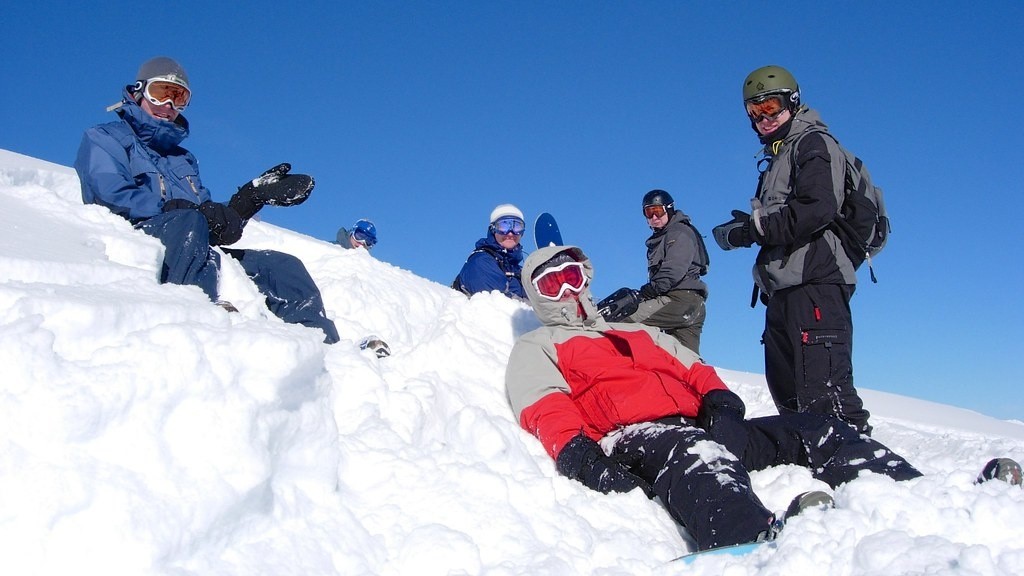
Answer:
(230, 162), (315, 218)
(556, 433), (652, 498)
(635, 283), (657, 302)
(699, 387), (751, 456)
(712, 209), (752, 251)
(162, 196), (244, 246)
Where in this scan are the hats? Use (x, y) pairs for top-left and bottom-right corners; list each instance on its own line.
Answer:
(138, 56), (189, 90)
(490, 204), (524, 222)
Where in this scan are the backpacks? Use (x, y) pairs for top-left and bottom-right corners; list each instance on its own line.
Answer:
(789, 127), (891, 272)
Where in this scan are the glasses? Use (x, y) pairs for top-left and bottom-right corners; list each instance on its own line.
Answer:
(533, 263), (588, 302)
(642, 205), (667, 218)
(492, 218), (525, 235)
(139, 77), (193, 111)
(352, 229), (376, 248)
(744, 96), (789, 122)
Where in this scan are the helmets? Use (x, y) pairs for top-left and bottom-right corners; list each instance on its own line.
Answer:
(643, 189), (675, 209)
(742, 66), (798, 96)
(354, 220), (376, 237)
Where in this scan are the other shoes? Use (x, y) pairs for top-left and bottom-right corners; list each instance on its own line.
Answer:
(975, 454), (1022, 487)
(769, 488), (836, 541)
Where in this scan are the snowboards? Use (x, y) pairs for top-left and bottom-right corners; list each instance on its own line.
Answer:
(533, 212), (564, 250)
(668, 539), (776, 565)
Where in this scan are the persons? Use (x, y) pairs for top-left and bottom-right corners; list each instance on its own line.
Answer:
(505, 246), (1022, 552)
(74, 56), (390, 358)
(713, 66), (890, 437)
(329, 219), (378, 252)
(452, 203), (534, 303)
(620, 190), (710, 355)
